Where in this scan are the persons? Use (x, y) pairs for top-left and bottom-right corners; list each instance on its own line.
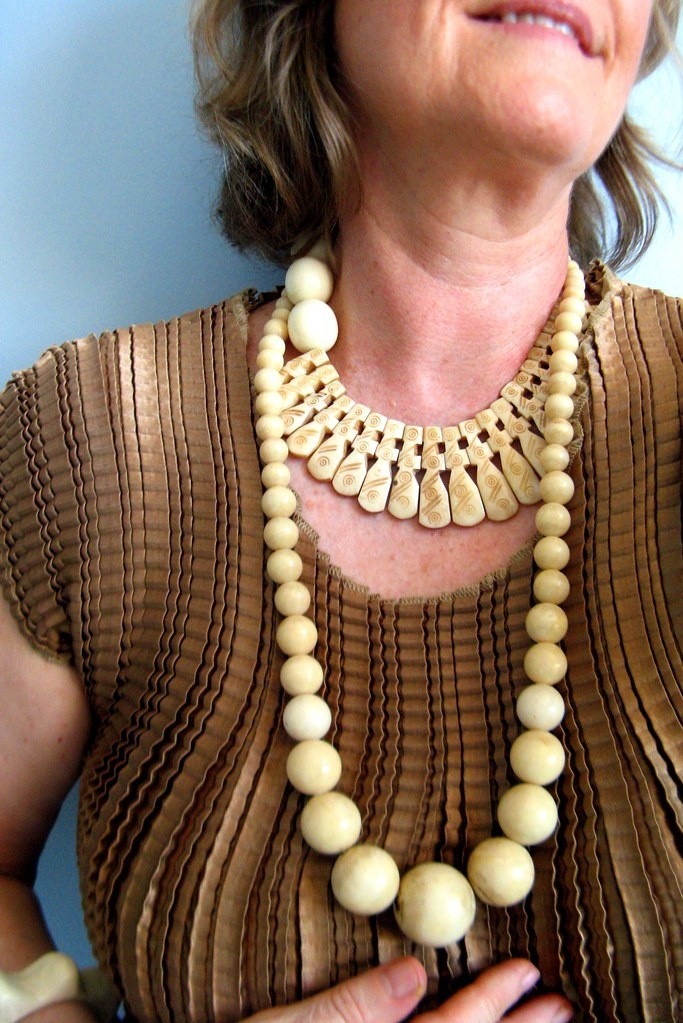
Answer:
(0, 0), (683, 1023)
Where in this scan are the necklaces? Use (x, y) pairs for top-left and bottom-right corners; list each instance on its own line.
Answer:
(253, 231), (584, 944)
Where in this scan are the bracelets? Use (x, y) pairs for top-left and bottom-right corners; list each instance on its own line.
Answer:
(0, 952), (124, 1023)
